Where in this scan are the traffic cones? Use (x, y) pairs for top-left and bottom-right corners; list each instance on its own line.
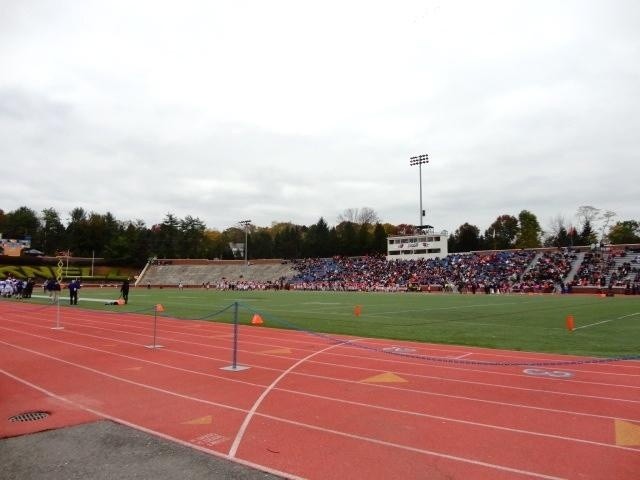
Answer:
(566, 315), (576, 330)
(353, 304), (362, 317)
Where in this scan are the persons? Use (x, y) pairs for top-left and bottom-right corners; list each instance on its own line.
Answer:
(121, 280), (129, 305)
(146, 280), (150, 288)
(0, 276), (34, 300)
(68, 280), (81, 305)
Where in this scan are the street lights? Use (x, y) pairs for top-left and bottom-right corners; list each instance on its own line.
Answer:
(239, 219), (252, 265)
(410, 152), (429, 235)
